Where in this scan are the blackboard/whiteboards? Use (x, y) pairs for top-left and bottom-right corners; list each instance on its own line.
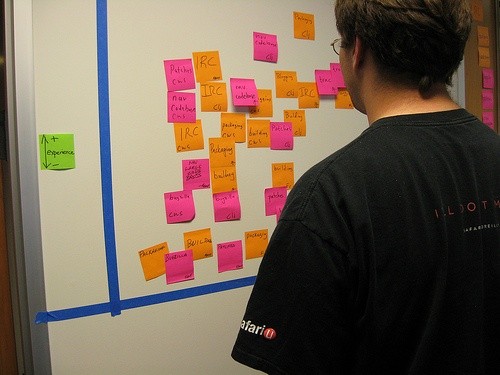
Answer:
(4, 0), (466, 375)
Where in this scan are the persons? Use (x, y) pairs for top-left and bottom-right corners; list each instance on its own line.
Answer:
(231, 0), (500, 375)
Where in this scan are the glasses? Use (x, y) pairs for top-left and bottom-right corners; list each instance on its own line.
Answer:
(329, 36), (344, 55)
